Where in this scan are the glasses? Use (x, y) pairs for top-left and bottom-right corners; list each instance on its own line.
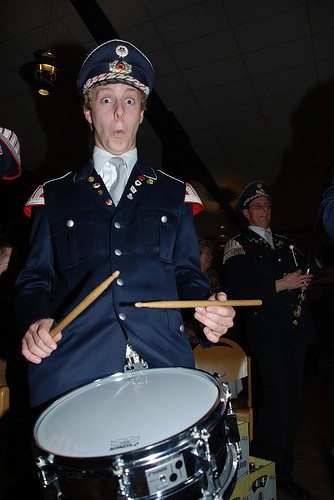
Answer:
(246, 203), (270, 210)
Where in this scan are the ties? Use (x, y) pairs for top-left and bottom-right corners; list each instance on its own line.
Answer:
(107, 157), (127, 207)
(264, 231), (274, 247)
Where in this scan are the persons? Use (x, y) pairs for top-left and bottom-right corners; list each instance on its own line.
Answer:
(13, 38), (235, 423)
(0, 127), (334, 500)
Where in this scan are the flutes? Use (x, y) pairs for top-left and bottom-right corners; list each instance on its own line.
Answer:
(292, 264), (312, 326)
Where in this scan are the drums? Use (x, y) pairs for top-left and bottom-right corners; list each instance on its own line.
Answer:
(31, 366), (241, 500)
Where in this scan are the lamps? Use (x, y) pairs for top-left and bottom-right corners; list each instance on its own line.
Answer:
(31, 0), (59, 98)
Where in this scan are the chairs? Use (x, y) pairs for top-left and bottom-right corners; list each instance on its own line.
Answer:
(193, 338), (254, 443)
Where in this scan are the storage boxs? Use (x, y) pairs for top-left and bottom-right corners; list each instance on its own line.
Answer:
(229, 420), (277, 500)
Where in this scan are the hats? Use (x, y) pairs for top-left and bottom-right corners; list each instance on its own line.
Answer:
(76, 39), (156, 99)
(236, 180), (271, 212)
